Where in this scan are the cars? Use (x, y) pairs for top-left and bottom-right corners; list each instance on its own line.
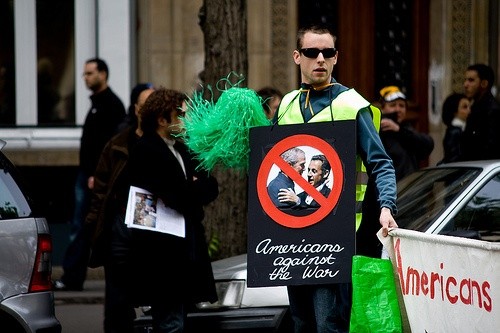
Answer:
(186, 159), (500, 333)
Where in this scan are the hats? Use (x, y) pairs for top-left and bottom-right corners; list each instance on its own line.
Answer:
(379, 85), (407, 101)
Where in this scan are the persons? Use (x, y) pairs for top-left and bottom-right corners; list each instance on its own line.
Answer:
(265, 26), (400, 333)
(52, 58), (128, 291)
(356, 86), (434, 259)
(436, 61), (500, 208)
(93, 83), (226, 333)
(257, 87), (284, 120)
(267, 147), (332, 217)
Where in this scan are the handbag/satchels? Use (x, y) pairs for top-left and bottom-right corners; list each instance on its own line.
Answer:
(68, 218), (107, 268)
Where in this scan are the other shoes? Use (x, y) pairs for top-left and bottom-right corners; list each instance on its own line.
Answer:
(53, 280), (82, 290)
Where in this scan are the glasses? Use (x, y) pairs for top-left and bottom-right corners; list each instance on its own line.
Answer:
(297, 47), (337, 59)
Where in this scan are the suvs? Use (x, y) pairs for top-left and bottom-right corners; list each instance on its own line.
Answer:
(0, 139), (63, 333)
